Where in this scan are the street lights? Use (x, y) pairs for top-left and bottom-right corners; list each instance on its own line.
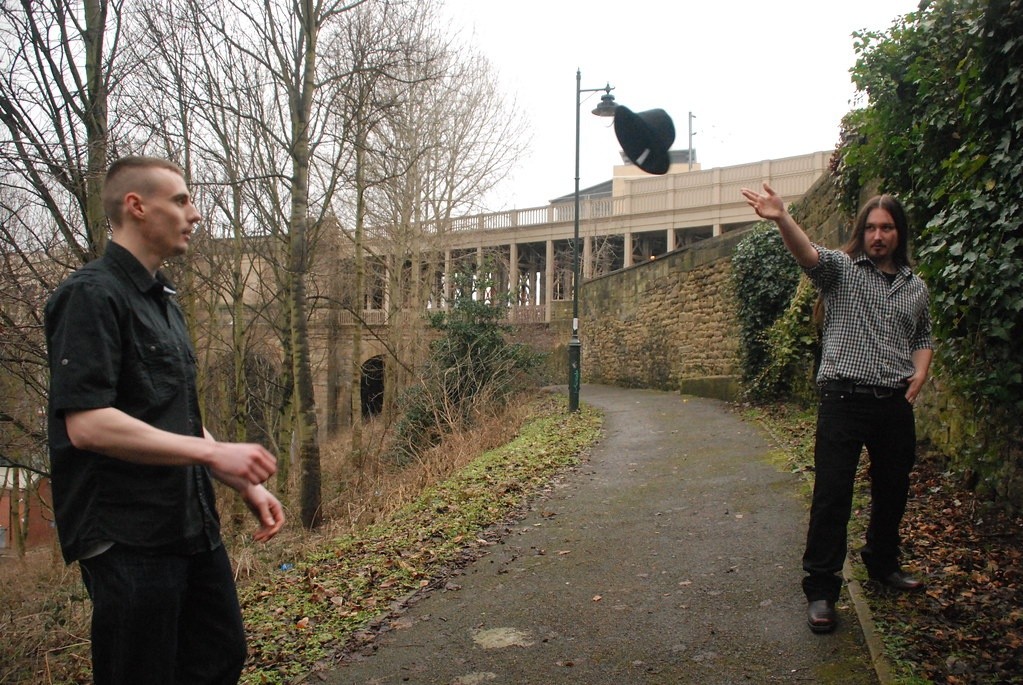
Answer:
(566, 69), (622, 413)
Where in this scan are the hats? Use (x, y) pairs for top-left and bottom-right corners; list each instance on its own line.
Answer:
(613, 105), (675, 175)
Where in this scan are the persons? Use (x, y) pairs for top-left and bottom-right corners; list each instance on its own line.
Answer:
(739, 179), (935, 632)
(40, 153), (287, 685)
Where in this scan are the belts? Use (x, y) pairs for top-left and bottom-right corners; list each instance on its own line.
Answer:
(824, 381), (906, 395)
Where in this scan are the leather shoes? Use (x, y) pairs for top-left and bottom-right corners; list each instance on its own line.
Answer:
(807, 596), (837, 631)
(869, 570), (926, 593)
